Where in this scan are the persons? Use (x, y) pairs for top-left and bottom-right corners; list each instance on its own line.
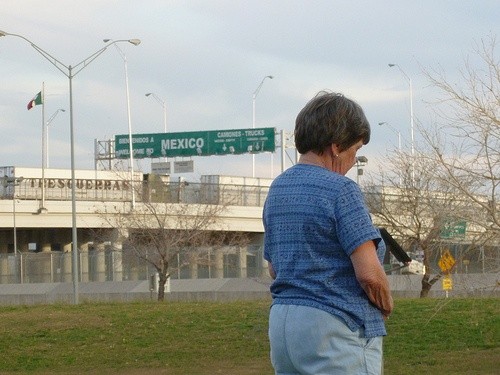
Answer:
(263, 91), (394, 375)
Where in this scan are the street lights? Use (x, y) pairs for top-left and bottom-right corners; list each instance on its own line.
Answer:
(376, 121), (402, 188)
(40, 108), (67, 210)
(388, 64), (416, 189)
(0, 29), (142, 305)
(250, 75), (274, 179)
(146, 92), (170, 132)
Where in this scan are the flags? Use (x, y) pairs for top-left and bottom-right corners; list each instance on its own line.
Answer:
(27, 91), (42, 110)
